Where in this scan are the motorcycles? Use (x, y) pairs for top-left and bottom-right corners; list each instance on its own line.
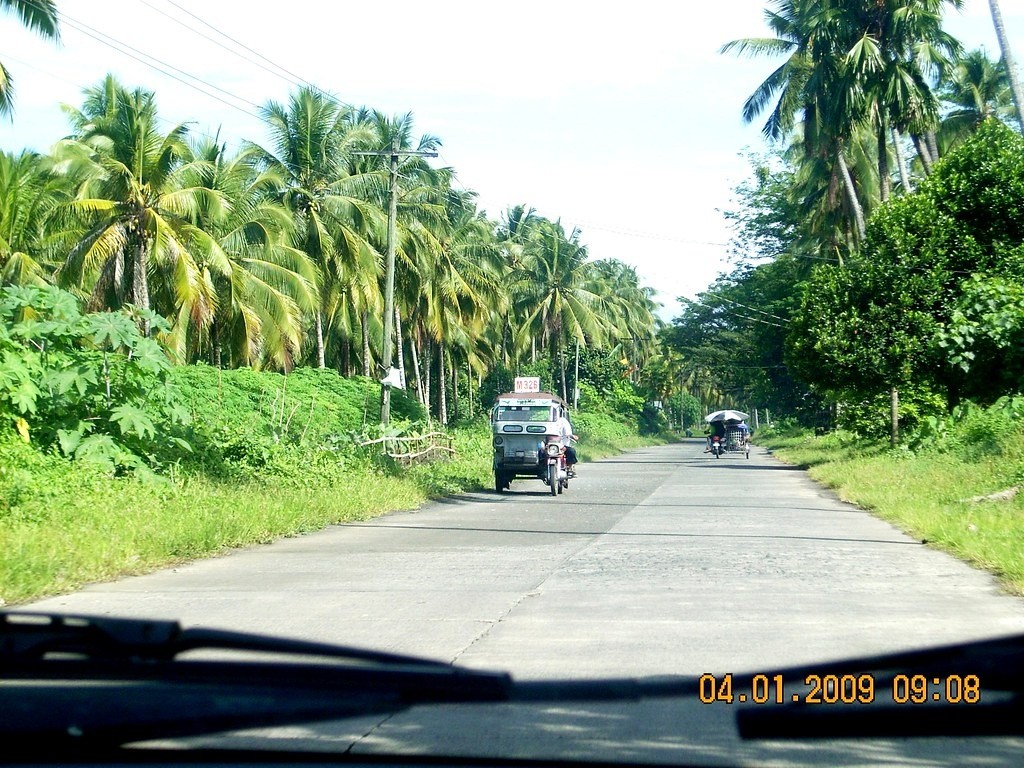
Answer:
(703, 429), (727, 459)
(538, 431), (579, 496)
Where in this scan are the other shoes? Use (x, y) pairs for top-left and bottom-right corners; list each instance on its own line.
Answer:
(703, 449), (711, 453)
(568, 471), (577, 477)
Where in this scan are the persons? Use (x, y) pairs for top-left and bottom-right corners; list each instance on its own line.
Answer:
(703, 420), (751, 453)
(537, 407), (577, 476)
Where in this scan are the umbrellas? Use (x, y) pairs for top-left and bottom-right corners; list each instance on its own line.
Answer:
(705, 409), (751, 423)
